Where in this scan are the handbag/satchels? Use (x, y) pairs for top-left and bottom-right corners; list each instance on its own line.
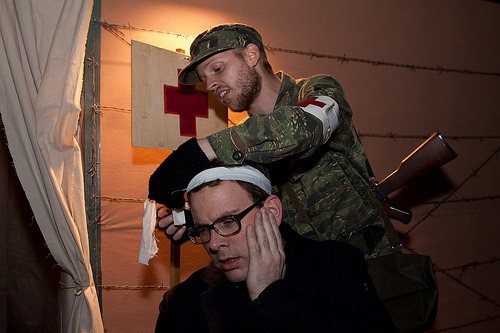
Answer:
(366, 252), (439, 333)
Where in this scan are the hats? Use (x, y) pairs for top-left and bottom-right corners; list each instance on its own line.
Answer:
(178, 23), (262, 85)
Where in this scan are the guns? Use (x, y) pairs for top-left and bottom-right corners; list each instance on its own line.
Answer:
(368, 133), (456, 223)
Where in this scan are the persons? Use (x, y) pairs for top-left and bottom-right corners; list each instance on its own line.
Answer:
(148, 23), (440, 333)
(155, 159), (398, 333)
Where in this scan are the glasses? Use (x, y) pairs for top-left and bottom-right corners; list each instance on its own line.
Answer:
(185, 195), (267, 244)
